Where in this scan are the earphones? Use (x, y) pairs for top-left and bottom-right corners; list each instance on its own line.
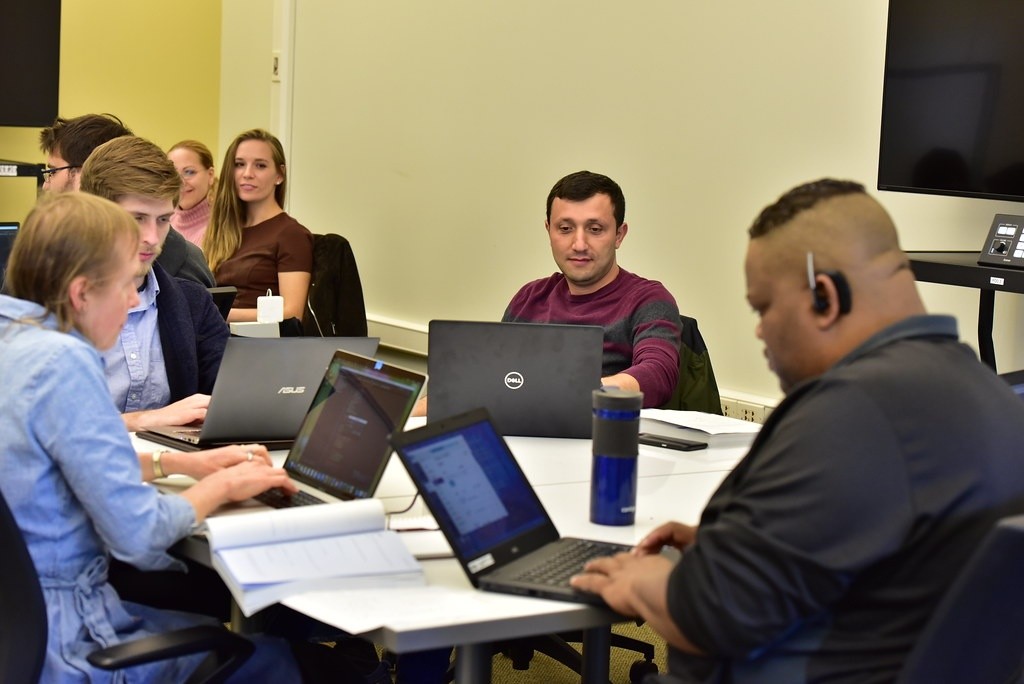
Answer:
(806, 252), (832, 314)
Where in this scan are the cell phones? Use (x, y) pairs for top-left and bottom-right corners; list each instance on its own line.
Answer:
(638, 433), (707, 451)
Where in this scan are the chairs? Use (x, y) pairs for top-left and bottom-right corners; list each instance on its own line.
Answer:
(295, 233), (368, 336)
(483, 316), (719, 684)
(886, 512), (1024, 684)
(0, 495), (255, 684)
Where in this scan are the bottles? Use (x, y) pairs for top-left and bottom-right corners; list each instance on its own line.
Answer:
(590, 385), (643, 525)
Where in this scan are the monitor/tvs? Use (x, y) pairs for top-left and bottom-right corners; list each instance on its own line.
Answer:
(879, 0), (1024, 203)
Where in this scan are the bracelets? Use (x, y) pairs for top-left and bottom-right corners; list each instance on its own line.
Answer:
(153, 450), (169, 477)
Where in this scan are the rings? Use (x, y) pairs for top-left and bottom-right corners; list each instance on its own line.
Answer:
(248, 453), (253, 461)
(241, 445), (244, 447)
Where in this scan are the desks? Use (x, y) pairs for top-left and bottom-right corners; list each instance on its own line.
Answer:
(127, 406), (765, 684)
(902, 251), (1024, 383)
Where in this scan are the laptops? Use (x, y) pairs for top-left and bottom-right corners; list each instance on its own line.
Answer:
(134, 321), (638, 607)
(0, 222), (22, 289)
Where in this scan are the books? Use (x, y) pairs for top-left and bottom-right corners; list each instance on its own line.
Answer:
(196, 498), (424, 619)
(639, 409), (763, 448)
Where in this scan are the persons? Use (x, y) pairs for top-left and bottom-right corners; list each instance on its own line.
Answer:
(0, 192), (357, 684)
(413, 171), (682, 417)
(167, 140), (216, 249)
(203, 129), (311, 322)
(77, 136), (230, 433)
(40, 113), (132, 192)
(569, 180), (1024, 684)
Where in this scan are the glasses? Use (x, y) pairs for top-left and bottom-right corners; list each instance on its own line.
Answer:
(177, 168), (208, 180)
(40, 165), (81, 182)
